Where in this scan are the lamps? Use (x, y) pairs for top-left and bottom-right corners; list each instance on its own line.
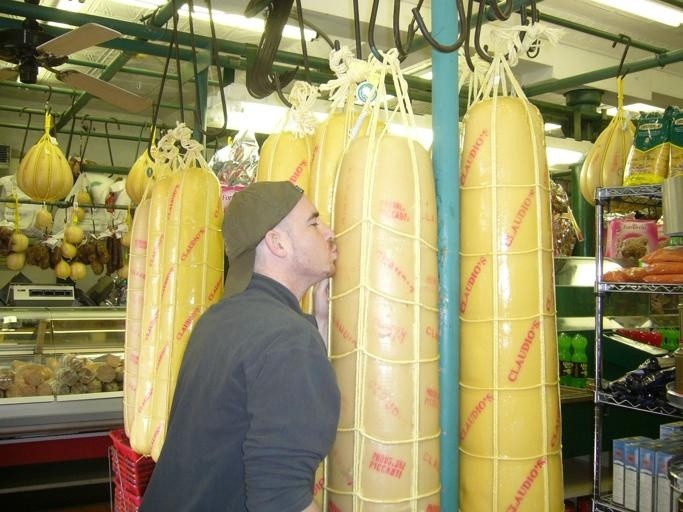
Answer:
(203, 64), (599, 177)
(587, 0), (683, 28)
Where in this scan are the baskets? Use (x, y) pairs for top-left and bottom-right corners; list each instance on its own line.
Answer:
(110, 427), (158, 511)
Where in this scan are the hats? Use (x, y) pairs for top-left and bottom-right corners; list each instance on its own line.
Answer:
(220, 180), (304, 301)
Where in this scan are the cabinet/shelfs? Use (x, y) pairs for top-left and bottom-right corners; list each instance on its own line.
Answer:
(590, 178), (683, 512)
(0, 305), (128, 512)
(556, 314), (654, 512)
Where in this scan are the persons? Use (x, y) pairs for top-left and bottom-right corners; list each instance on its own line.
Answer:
(137, 181), (340, 512)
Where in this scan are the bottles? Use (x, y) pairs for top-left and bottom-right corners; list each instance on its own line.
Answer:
(558, 332), (588, 388)
(673, 336), (682, 393)
(616, 329), (681, 354)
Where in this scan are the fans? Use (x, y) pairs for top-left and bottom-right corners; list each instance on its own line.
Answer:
(0, 0), (153, 114)
